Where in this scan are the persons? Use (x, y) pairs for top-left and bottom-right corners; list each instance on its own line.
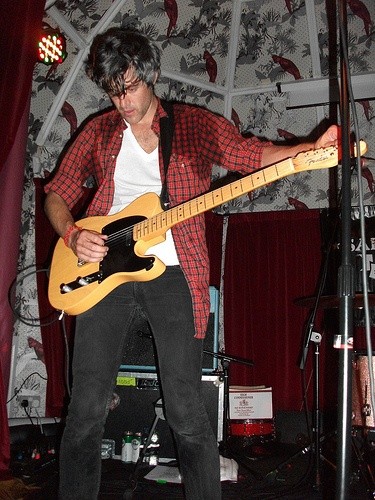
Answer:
(43, 26), (353, 500)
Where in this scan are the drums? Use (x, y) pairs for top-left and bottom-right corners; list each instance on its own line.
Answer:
(345, 352), (375, 439)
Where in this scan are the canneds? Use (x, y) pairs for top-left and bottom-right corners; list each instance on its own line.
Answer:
(122, 431), (142, 463)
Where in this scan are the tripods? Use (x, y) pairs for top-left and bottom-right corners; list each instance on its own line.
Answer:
(262, 308), (375, 500)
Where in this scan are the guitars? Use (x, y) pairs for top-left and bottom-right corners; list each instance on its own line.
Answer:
(47, 136), (371, 317)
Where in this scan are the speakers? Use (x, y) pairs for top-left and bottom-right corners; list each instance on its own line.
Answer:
(118, 285), (219, 373)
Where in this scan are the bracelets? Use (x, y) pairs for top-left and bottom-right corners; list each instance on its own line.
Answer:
(63, 224), (78, 247)
